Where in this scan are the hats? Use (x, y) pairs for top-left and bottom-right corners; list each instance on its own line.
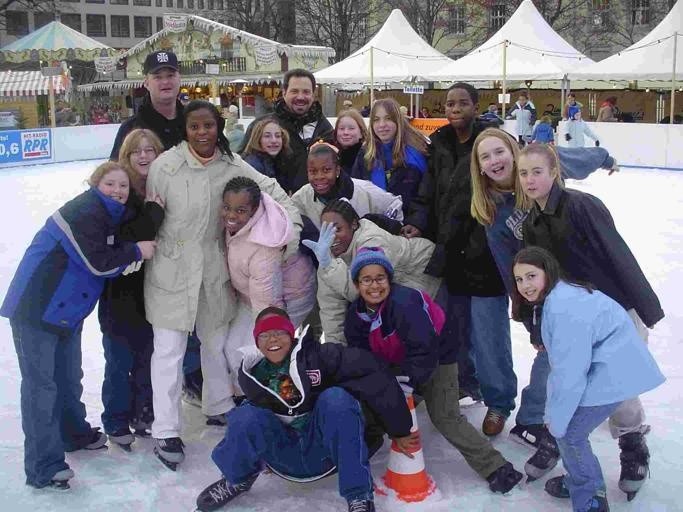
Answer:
(350, 246), (396, 282)
(140, 50), (180, 74)
(568, 107), (581, 119)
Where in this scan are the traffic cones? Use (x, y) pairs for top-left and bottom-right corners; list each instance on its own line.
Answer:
(370, 390), (440, 499)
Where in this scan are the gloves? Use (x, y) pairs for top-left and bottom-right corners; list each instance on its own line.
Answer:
(594, 141), (600, 146)
(301, 220), (336, 268)
(121, 258), (142, 276)
(281, 224), (301, 265)
(604, 155), (620, 176)
(566, 134), (572, 141)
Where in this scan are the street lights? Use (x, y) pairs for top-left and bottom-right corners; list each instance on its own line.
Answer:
(226, 78), (248, 119)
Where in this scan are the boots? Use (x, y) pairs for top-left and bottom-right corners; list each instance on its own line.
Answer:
(621, 433), (654, 492)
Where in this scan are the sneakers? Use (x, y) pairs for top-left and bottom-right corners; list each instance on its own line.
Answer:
(154, 427), (186, 463)
(510, 418), (562, 459)
(525, 430), (565, 478)
(22, 457), (76, 483)
(62, 426), (107, 451)
(483, 403), (506, 436)
(129, 414), (150, 437)
(347, 492), (376, 511)
(205, 401), (236, 423)
(579, 489), (610, 512)
(488, 464), (525, 494)
(197, 468), (255, 511)
(544, 472), (571, 497)
(181, 384), (203, 406)
(101, 422), (136, 444)
